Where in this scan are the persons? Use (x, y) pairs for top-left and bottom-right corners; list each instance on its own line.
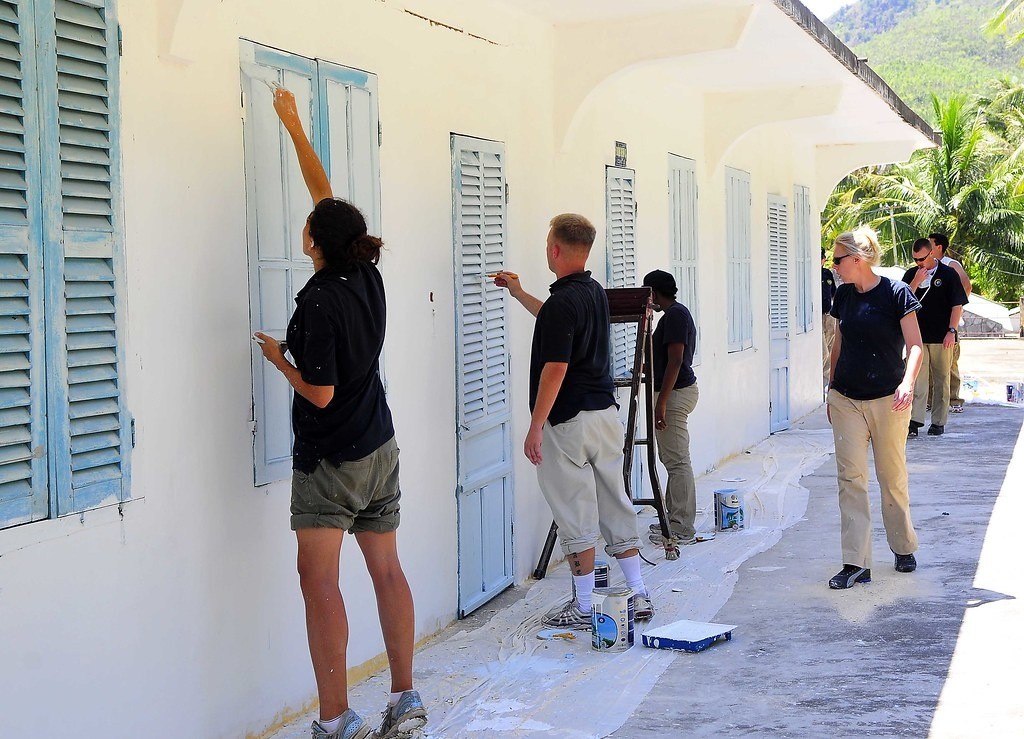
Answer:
(492, 213), (656, 629)
(641, 272), (699, 544)
(902, 233), (972, 439)
(253, 85), (426, 738)
(821, 245), (838, 402)
(825, 227), (925, 589)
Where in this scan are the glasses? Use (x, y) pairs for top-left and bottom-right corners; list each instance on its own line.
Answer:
(832, 254), (852, 265)
(913, 249), (932, 261)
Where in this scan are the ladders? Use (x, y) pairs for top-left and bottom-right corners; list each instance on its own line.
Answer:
(534, 288), (677, 580)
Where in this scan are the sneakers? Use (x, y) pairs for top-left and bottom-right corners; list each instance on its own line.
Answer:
(649, 533), (697, 546)
(370, 691), (427, 739)
(633, 591), (655, 619)
(894, 553), (917, 573)
(310, 708), (374, 739)
(540, 597), (592, 632)
(926, 404), (931, 411)
(829, 565), (871, 589)
(649, 523), (663, 534)
(952, 405), (964, 413)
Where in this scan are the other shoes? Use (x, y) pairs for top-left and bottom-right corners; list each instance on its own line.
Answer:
(907, 427), (918, 439)
(927, 423), (944, 435)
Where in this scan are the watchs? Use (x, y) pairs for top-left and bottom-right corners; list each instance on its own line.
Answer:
(948, 326), (957, 333)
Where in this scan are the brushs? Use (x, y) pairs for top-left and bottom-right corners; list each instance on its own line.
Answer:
(474, 273), (518, 280)
(241, 59), (272, 91)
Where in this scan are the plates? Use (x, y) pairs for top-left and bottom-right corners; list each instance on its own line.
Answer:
(537, 629), (578, 640)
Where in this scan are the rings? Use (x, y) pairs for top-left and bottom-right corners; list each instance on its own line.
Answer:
(658, 420), (661, 422)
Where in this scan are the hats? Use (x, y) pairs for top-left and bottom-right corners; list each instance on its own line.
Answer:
(643, 270), (676, 291)
(821, 247), (826, 259)
